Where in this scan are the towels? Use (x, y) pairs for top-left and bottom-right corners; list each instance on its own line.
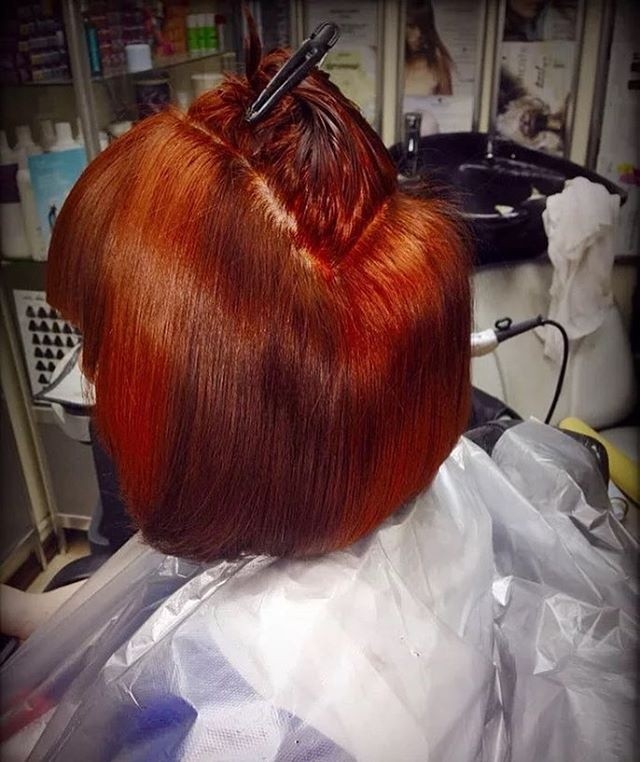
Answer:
(533, 175), (623, 364)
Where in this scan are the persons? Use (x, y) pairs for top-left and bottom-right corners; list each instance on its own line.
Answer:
(0, 51), (640, 762)
(405, 0), (452, 96)
(504, 0), (548, 41)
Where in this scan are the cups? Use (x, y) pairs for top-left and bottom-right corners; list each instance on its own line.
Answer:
(190, 73), (224, 98)
(130, 71), (172, 122)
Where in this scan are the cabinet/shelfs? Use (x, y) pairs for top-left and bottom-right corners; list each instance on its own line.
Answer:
(0, 0), (241, 555)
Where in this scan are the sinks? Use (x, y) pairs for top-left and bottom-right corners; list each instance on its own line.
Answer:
(388, 127), (629, 264)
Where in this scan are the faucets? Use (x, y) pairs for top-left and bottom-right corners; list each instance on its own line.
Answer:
(404, 111), (421, 178)
(485, 129), (517, 161)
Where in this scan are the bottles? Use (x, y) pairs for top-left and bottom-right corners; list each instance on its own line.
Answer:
(0, 115), (90, 262)
